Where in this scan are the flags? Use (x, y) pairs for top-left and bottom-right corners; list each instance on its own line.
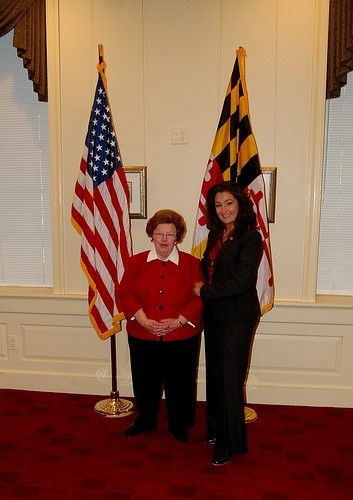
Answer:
(193, 48), (275, 316)
(71, 60), (133, 341)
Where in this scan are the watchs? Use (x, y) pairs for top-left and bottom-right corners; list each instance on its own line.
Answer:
(177, 320), (183, 327)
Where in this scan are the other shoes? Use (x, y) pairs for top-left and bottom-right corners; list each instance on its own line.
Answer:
(125, 423), (157, 437)
(168, 426), (189, 443)
(211, 452), (232, 465)
(202, 434), (217, 444)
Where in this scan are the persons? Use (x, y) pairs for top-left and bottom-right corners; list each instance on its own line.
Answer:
(119, 209), (206, 442)
(195, 181), (263, 466)
